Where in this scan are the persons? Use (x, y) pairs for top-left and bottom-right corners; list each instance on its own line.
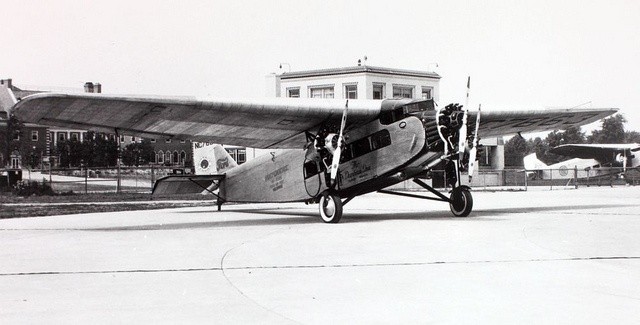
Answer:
(616, 152), (621, 167)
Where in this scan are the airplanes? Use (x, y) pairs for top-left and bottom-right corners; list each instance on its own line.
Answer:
(523, 143), (640, 186)
(9, 91), (620, 224)
